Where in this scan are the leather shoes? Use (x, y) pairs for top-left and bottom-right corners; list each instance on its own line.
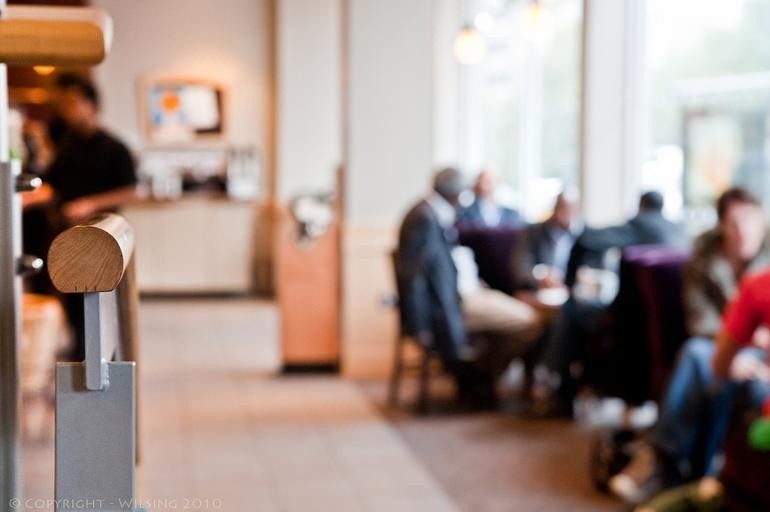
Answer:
(606, 473), (659, 506)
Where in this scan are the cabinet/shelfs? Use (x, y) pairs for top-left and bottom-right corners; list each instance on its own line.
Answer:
(110, 204), (256, 294)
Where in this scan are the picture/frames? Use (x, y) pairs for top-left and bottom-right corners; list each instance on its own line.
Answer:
(132, 70), (232, 150)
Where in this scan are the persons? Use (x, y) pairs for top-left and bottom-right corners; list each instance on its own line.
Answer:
(14, 70), (135, 364)
(465, 168), (768, 510)
(394, 165), (539, 416)
